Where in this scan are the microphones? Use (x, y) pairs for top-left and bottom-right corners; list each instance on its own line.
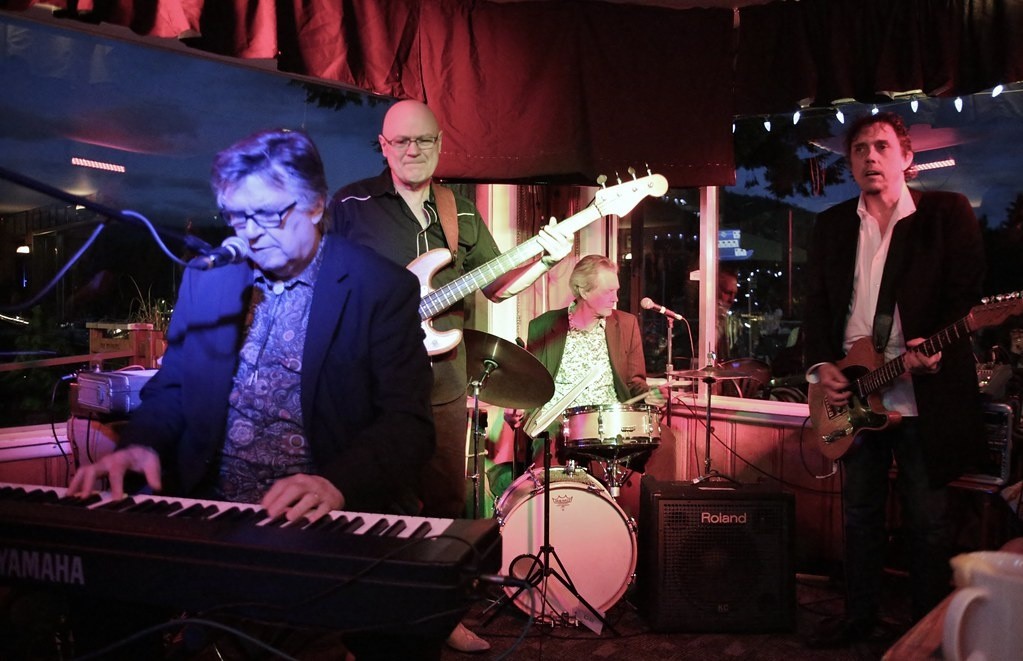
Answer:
(640, 297), (684, 320)
(195, 236), (249, 269)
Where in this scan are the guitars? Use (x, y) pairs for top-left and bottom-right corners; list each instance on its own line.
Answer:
(808, 291), (1023, 462)
(403, 160), (672, 357)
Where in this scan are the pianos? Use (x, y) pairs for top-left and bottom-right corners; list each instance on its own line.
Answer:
(0, 479), (503, 661)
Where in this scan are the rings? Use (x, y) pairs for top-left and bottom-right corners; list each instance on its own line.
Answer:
(312, 493), (320, 503)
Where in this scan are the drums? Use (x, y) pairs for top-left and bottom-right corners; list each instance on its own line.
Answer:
(492, 466), (637, 623)
(561, 402), (663, 453)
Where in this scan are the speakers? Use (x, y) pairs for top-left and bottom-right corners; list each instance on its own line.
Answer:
(635, 474), (798, 633)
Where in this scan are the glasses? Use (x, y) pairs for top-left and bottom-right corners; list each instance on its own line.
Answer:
(382, 133), (439, 149)
(219, 192), (305, 229)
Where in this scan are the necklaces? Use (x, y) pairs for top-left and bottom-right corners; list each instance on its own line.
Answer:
(249, 280), (284, 386)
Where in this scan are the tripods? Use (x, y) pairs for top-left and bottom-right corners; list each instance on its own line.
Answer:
(479, 364), (625, 639)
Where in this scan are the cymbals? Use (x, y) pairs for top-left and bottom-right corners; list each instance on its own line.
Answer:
(664, 367), (768, 382)
(459, 326), (556, 411)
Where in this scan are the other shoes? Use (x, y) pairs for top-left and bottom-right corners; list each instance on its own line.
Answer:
(806, 605), (879, 651)
(446, 622), (490, 652)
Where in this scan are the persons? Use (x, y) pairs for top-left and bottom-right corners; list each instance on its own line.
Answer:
(803, 111), (982, 649)
(64, 128), (435, 524)
(323, 102), (575, 653)
(482, 254), (668, 496)
(690, 268), (739, 369)
